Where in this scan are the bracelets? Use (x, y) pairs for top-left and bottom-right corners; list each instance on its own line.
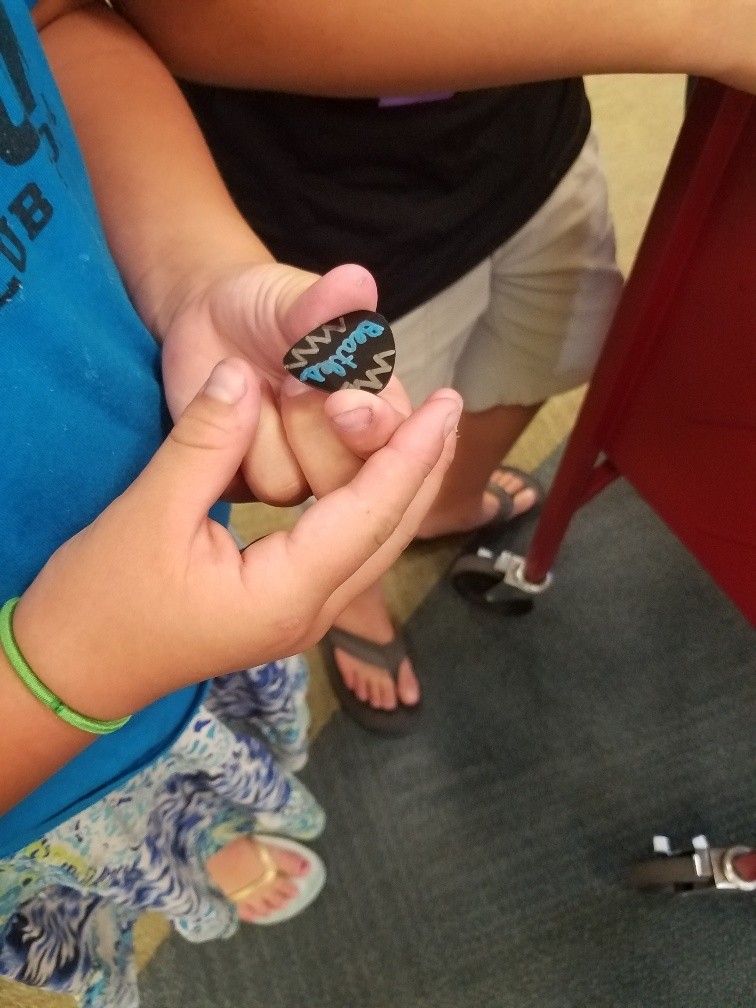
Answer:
(0, 596), (131, 736)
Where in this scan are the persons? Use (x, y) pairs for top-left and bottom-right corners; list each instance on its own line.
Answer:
(0, 0), (463, 1008)
(110, 0), (755, 735)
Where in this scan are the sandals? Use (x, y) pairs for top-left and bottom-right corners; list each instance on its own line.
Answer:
(226, 828), (327, 925)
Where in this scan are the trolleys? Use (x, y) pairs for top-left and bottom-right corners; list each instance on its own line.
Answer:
(448, 72), (756, 898)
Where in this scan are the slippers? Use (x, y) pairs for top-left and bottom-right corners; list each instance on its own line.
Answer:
(316, 624), (422, 737)
(405, 466), (544, 548)
